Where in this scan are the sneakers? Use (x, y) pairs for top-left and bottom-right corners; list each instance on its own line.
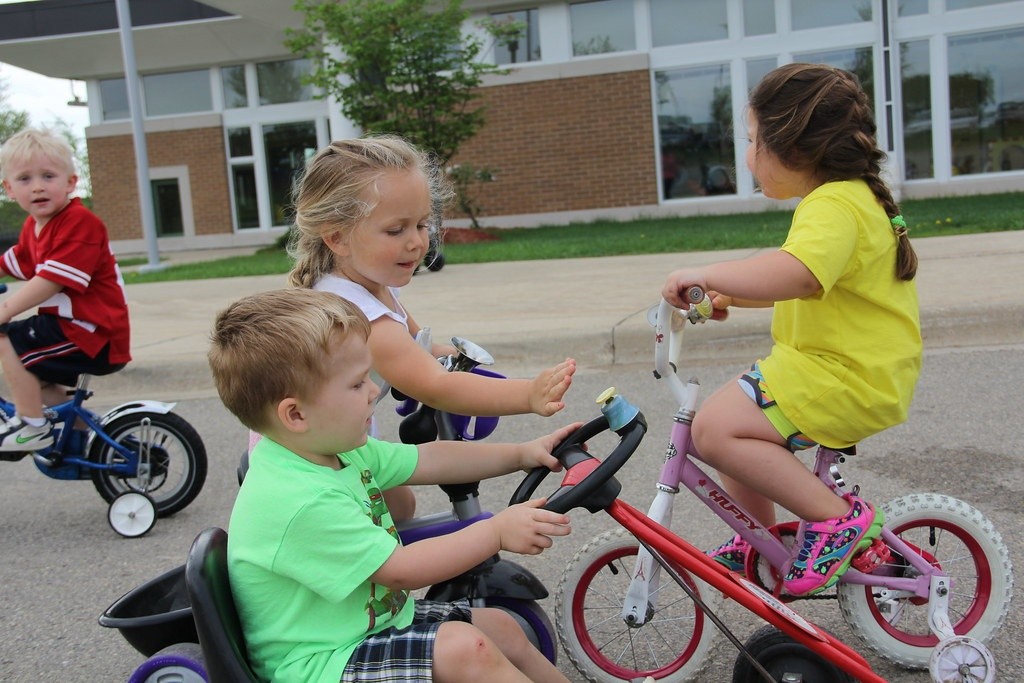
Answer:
(0, 416), (54, 451)
(783, 485), (885, 597)
(702, 535), (750, 576)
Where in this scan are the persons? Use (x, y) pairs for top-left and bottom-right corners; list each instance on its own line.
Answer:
(209, 289), (569, 682)
(0, 129), (132, 455)
(248, 137), (576, 524)
(662, 62), (921, 596)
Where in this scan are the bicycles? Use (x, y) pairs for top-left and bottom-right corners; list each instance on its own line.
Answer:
(0, 284), (209, 539)
(549, 286), (1013, 683)
